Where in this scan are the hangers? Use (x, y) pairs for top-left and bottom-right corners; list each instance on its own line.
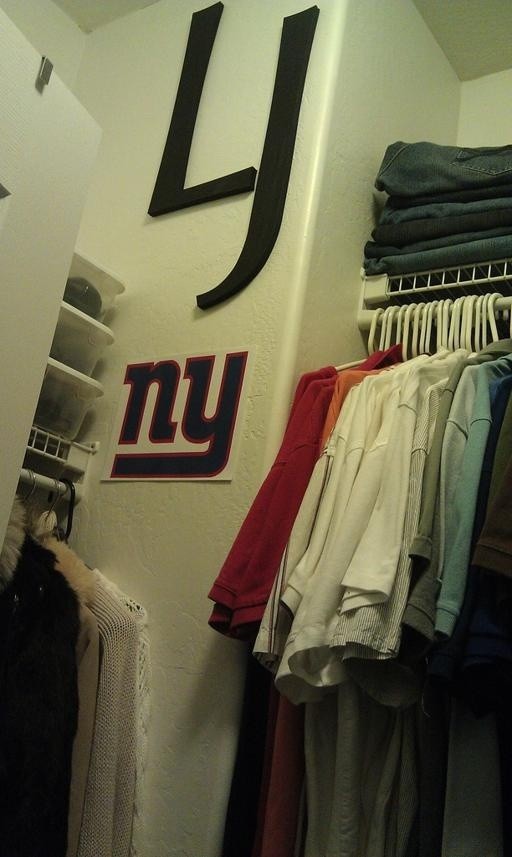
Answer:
(16, 467), (147, 616)
(333, 291), (510, 376)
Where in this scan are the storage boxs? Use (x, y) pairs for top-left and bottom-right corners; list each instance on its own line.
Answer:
(63, 246), (130, 324)
(33, 356), (105, 442)
(49, 301), (117, 377)
